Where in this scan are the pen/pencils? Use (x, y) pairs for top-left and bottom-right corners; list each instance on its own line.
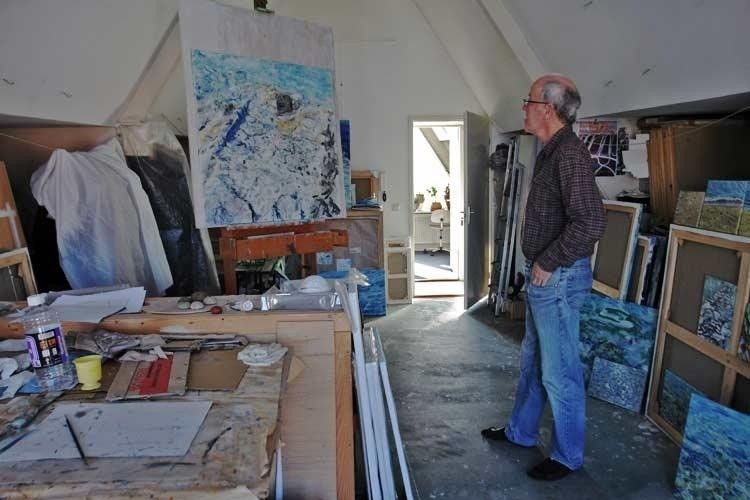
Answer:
(64, 413), (88, 466)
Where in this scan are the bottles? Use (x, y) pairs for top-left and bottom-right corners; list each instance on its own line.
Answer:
(23, 293), (74, 392)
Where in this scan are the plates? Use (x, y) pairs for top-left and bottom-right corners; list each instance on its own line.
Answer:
(140, 296), (226, 315)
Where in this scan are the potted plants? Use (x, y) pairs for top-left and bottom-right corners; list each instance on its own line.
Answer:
(429, 186), (442, 211)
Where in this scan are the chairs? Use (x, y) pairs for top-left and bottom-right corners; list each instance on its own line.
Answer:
(423, 208), (450, 256)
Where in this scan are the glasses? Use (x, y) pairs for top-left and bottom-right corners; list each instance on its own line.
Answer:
(522, 95), (559, 111)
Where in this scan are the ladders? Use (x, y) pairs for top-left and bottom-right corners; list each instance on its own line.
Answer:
(487, 136), (526, 318)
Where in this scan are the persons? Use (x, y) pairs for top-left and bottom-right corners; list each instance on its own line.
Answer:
(481, 74), (607, 481)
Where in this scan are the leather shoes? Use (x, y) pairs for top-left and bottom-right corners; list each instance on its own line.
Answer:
(481, 425), (537, 449)
(525, 456), (570, 482)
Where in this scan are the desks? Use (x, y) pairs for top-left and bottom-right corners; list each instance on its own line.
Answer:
(1, 292), (364, 500)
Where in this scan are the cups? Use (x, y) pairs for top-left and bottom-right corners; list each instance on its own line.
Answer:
(70, 354), (104, 392)
